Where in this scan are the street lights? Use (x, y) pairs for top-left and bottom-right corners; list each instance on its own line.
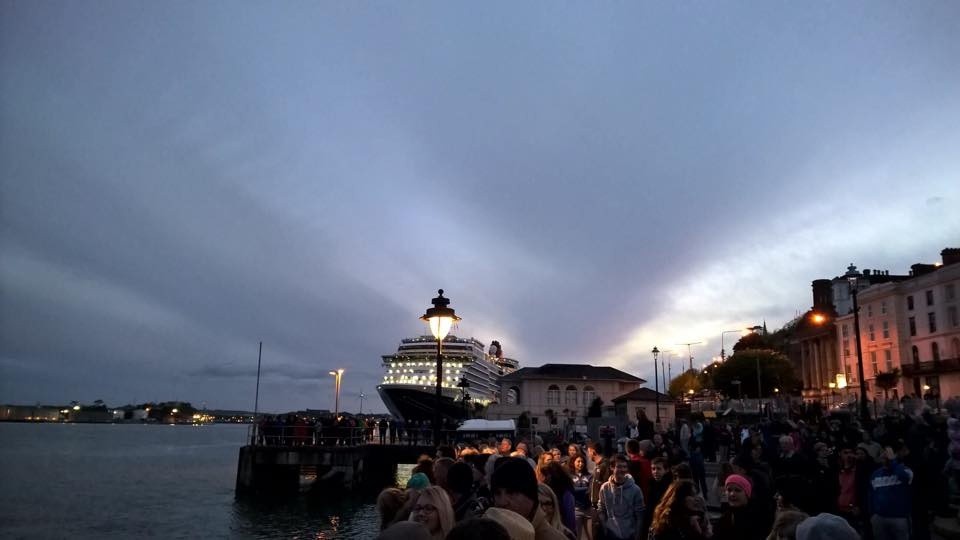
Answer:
(327, 369), (338, 419)
(419, 289), (463, 444)
(651, 346), (661, 423)
(721, 329), (748, 363)
(810, 287), (870, 422)
(673, 341), (703, 369)
(359, 393), (364, 413)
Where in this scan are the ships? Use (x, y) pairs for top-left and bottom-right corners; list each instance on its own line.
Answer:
(375, 331), (519, 428)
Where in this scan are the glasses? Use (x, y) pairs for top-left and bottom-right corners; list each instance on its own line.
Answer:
(413, 503), (437, 514)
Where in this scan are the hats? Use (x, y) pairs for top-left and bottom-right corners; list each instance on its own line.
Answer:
(725, 473), (752, 498)
(490, 458), (537, 501)
(796, 512), (861, 540)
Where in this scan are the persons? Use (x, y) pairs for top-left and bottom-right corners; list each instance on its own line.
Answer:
(255, 399), (960, 540)
